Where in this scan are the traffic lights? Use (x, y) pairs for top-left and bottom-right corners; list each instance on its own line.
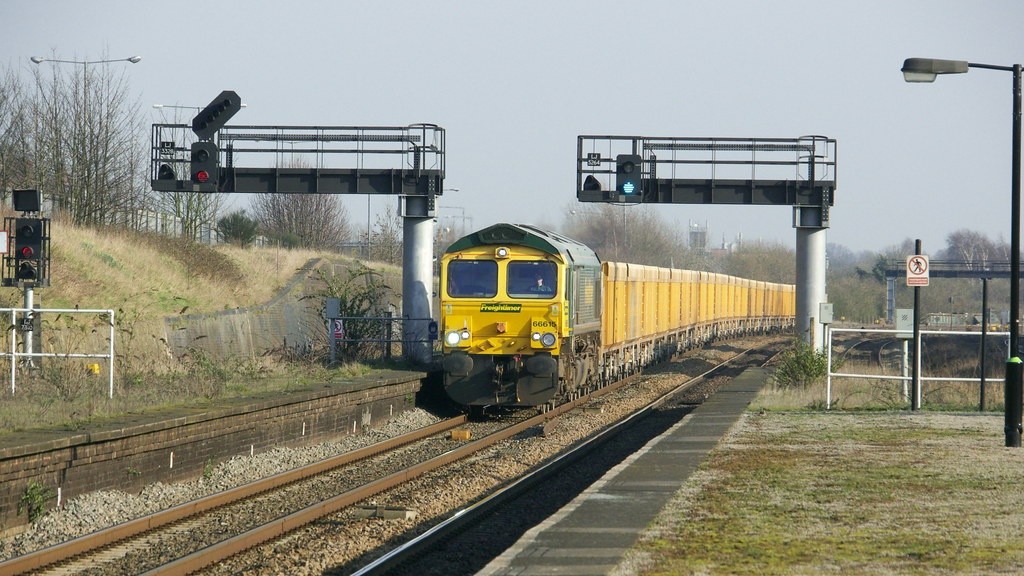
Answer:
(192, 90), (241, 140)
(191, 142), (217, 183)
(14, 217), (42, 261)
(17, 261), (37, 280)
(616, 154), (642, 197)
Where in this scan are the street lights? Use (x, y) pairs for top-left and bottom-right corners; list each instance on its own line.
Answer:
(31, 55), (142, 223)
(901, 57), (1024, 438)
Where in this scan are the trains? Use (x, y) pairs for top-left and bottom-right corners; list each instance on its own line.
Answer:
(437, 221), (796, 424)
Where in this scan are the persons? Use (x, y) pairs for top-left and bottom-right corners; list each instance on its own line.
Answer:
(528, 272), (551, 291)
